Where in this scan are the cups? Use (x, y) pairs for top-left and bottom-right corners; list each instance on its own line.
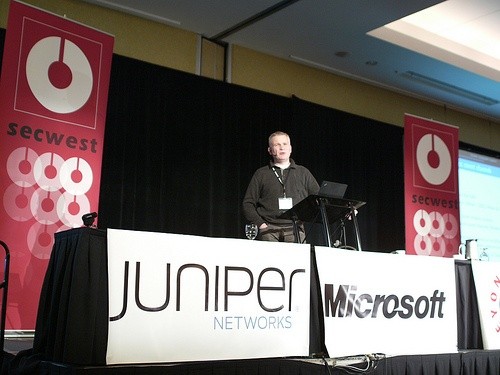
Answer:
(245, 224), (258, 240)
(458, 239), (479, 260)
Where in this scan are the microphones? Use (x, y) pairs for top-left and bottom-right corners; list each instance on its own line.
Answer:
(273, 154), (277, 156)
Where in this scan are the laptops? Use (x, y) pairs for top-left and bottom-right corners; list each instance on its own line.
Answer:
(318, 181), (348, 198)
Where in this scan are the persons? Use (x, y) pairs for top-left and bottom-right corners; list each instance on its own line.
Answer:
(242, 131), (358, 244)
